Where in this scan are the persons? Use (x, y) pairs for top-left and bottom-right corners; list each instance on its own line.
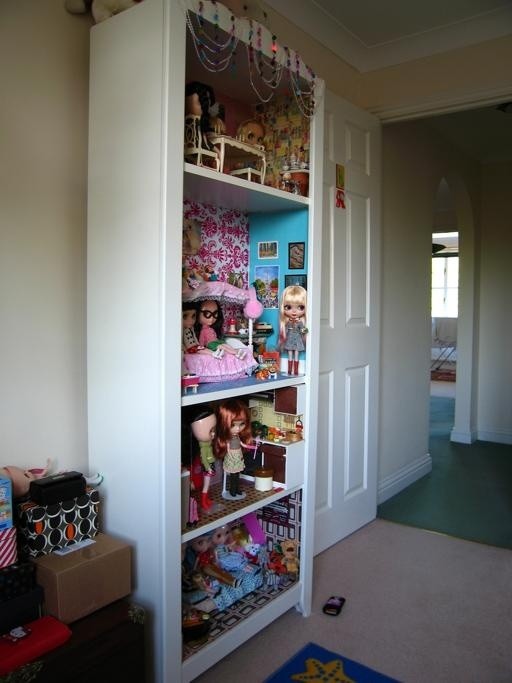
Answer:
(193, 524), (261, 588)
(180, 79), (310, 646)
(190, 399), (257, 514)
(235, 119), (264, 177)
(185, 80), (228, 179)
(182, 299), (246, 359)
(279, 285), (308, 375)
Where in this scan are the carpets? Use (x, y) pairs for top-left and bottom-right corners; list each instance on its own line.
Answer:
(262, 641), (404, 683)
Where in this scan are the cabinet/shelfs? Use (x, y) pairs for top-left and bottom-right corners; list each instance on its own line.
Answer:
(86, 1), (324, 683)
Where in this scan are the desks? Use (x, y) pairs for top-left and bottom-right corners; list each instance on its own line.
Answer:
(1, 589), (152, 683)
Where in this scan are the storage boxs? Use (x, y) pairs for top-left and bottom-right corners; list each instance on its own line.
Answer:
(0, 470), (133, 623)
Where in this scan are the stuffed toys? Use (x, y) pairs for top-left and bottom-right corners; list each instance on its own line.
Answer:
(0, 455), (105, 499)
